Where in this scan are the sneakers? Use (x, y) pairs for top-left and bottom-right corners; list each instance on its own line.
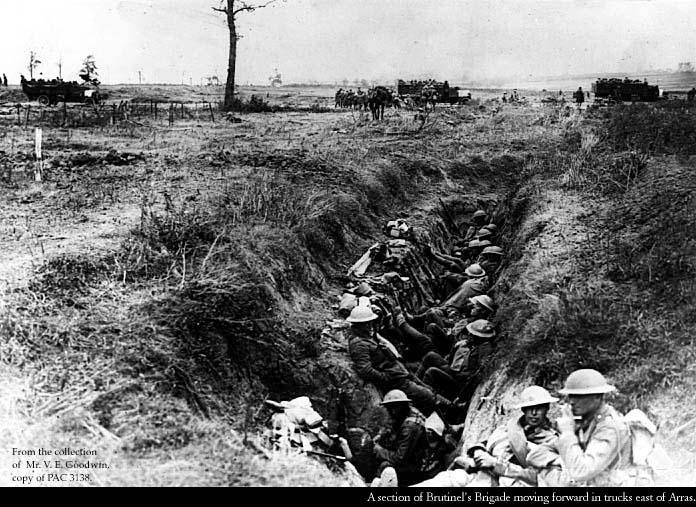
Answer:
(370, 477), (381, 487)
(380, 467), (397, 487)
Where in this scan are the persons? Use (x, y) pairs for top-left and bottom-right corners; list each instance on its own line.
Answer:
(336, 77), (441, 112)
(442, 80), (450, 103)
(344, 205), (507, 482)
(368, 380), (560, 489)
(574, 85), (585, 109)
(520, 365), (634, 486)
(500, 90), (508, 104)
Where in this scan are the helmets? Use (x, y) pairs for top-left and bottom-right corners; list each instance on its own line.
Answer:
(379, 389), (412, 405)
(464, 223), (505, 338)
(471, 210), (487, 219)
(512, 386), (559, 410)
(557, 368), (615, 396)
(345, 306), (378, 323)
